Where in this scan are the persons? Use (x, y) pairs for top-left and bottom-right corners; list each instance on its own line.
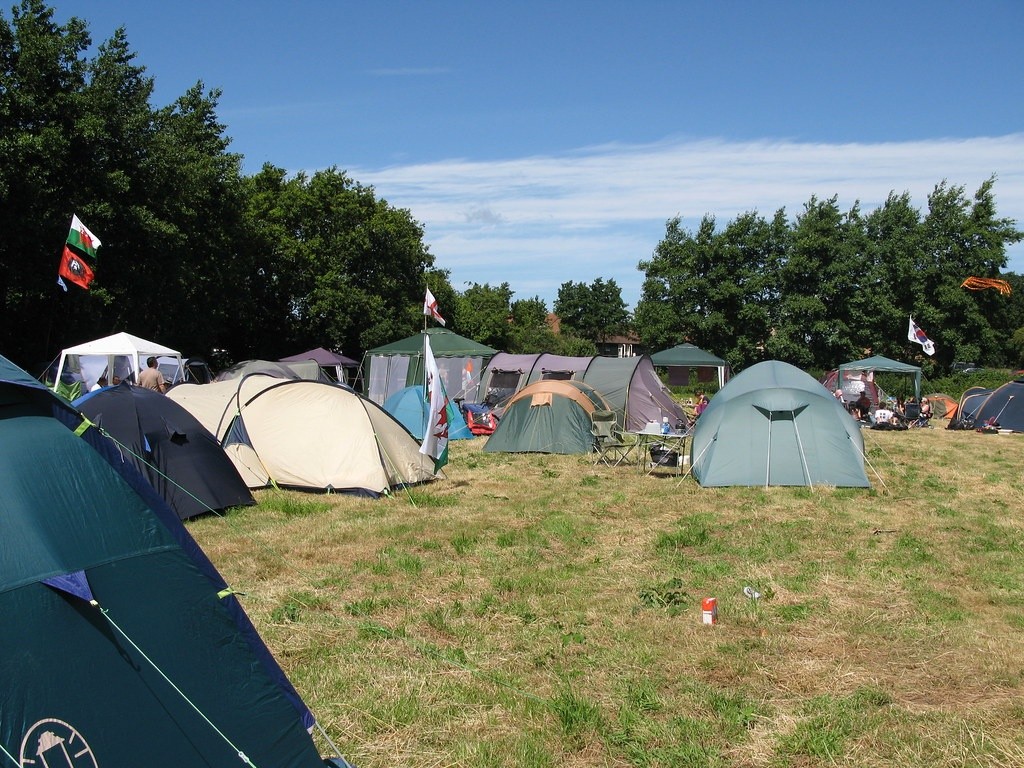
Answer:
(830, 389), (929, 430)
(688, 390), (711, 425)
(136, 356), (172, 395)
(91, 374), (120, 395)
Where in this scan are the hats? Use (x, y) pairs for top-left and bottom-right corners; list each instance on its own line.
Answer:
(860, 391), (865, 395)
(921, 397), (927, 402)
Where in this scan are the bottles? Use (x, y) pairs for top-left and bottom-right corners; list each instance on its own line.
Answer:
(742, 586), (760, 600)
(660, 416), (670, 434)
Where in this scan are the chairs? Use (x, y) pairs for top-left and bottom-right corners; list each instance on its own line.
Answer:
(590, 410), (636, 467)
(904, 402), (928, 429)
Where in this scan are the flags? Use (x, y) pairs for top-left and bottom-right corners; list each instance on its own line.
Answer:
(908, 314), (935, 356)
(423, 286), (446, 327)
(59, 245), (94, 290)
(418, 335), (455, 476)
(65, 212), (102, 259)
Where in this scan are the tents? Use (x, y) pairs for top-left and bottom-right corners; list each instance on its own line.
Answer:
(278, 347), (364, 393)
(819, 369), (880, 410)
(69, 379), (258, 522)
(922, 393), (959, 419)
(363, 327), (504, 419)
(2, 354), (352, 768)
(649, 342), (725, 390)
(53, 331), (186, 397)
(214, 357), (332, 385)
(481, 380), (626, 456)
(474, 351), (694, 436)
(380, 385), (475, 439)
(153, 356), (213, 384)
(838, 355), (921, 413)
(161, 373), (448, 500)
(689, 360), (871, 489)
(949, 386), (998, 426)
(974, 376), (1024, 432)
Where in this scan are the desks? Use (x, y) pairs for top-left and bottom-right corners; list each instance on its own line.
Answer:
(634, 429), (689, 476)
